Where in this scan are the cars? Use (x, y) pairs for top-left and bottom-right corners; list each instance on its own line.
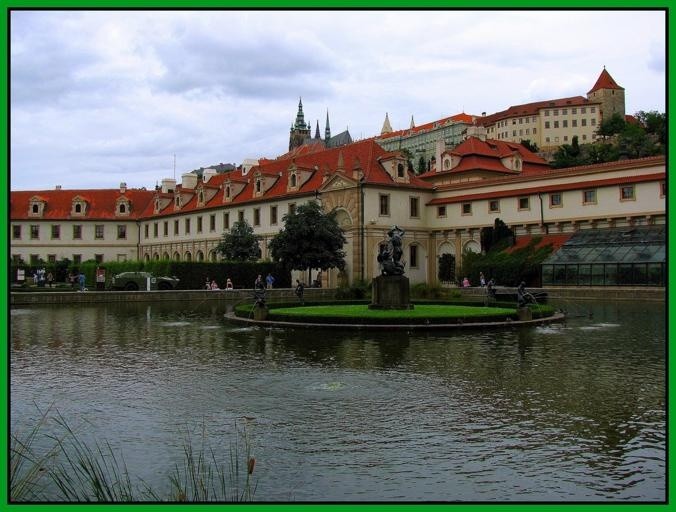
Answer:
(111, 271), (179, 291)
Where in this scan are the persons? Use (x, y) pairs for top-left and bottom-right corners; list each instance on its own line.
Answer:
(253, 274), (265, 290)
(210, 280), (218, 290)
(376, 244), (406, 273)
(517, 281), (528, 308)
(478, 272), (485, 288)
(205, 276), (211, 290)
(224, 278), (233, 291)
(65, 270), (86, 292)
(265, 273), (275, 289)
(462, 277), (471, 288)
(386, 225), (403, 268)
(486, 278), (497, 297)
(30, 266), (54, 288)
(294, 279), (304, 306)
